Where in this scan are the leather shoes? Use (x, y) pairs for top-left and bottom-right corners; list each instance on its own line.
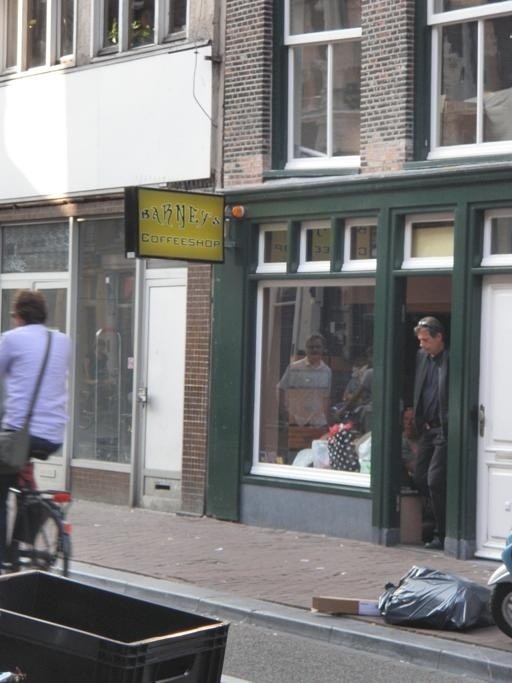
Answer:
(424, 535), (444, 548)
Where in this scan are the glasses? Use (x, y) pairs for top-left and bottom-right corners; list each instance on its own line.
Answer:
(418, 321), (435, 330)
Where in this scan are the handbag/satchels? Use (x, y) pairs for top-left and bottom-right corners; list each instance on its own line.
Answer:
(0, 424), (32, 476)
(328, 430), (361, 473)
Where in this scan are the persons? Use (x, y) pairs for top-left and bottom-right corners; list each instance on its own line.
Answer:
(1, 285), (71, 570)
(409, 314), (451, 549)
(401, 404), (418, 479)
(86, 336), (114, 411)
(272, 333), (373, 447)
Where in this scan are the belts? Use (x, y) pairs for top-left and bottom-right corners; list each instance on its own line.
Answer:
(420, 420), (440, 428)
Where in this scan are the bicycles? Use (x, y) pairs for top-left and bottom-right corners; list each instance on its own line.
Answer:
(0, 447), (72, 578)
